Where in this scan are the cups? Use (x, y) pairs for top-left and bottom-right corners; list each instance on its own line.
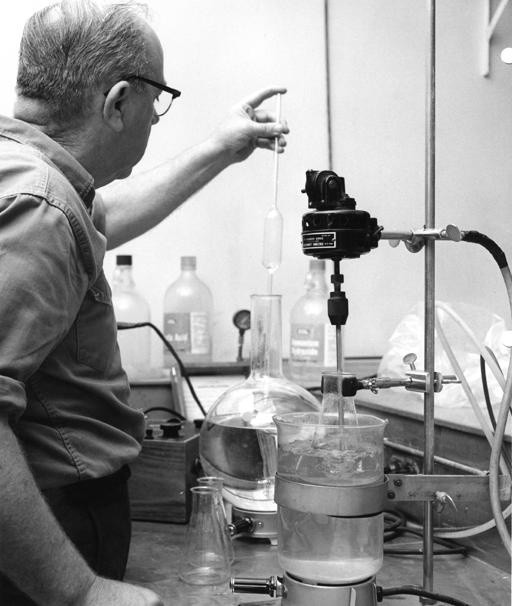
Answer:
(271, 407), (392, 586)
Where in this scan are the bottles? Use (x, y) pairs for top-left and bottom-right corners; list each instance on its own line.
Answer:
(176, 477), (235, 586)
(161, 254), (214, 369)
(196, 291), (327, 518)
(110, 253), (151, 383)
(288, 259), (338, 390)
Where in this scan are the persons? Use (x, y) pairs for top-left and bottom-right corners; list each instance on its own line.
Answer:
(0, 0), (292, 606)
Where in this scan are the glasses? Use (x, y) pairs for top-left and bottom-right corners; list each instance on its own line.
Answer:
(103, 74), (181, 116)
